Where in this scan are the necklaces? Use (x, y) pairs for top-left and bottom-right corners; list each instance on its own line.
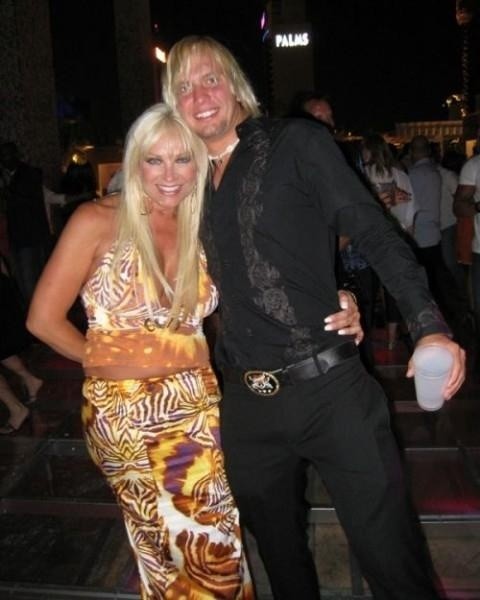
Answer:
(205, 139), (241, 159)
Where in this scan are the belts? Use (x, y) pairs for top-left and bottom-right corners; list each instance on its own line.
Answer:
(218, 340), (360, 398)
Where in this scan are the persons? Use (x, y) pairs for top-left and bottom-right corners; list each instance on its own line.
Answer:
(25, 102), (368, 598)
(300, 94), (480, 371)
(0, 253), (45, 437)
(159, 33), (466, 599)
(1, 140), (52, 301)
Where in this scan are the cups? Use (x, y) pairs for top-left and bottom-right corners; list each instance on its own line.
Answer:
(377, 183), (397, 207)
(412, 343), (454, 414)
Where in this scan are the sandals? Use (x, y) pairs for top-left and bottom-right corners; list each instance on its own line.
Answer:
(0, 411), (34, 438)
(22, 376), (48, 409)
